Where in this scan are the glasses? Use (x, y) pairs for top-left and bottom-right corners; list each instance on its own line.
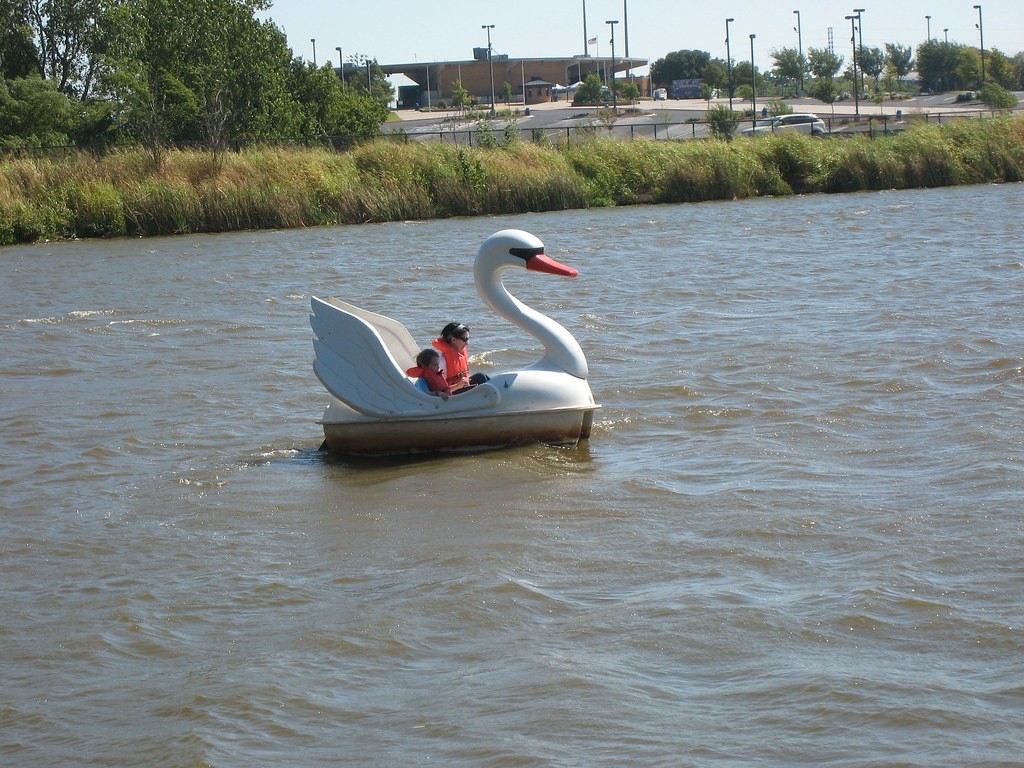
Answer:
(456, 335), (468, 342)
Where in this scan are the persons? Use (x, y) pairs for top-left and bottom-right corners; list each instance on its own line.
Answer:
(414, 349), (464, 401)
(406, 323), (491, 395)
(415, 102), (420, 110)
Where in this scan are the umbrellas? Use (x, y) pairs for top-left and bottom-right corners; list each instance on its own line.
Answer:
(552, 84), (566, 93)
(567, 80), (588, 88)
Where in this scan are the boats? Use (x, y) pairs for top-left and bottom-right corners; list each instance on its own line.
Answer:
(307, 228), (605, 453)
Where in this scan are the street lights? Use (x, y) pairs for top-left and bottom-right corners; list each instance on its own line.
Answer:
(335, 47), (344, 94)
(606, 20), (620, 113)
(749, 34), (756, 129)
(725, 17), (735, 113)
(844, 15), (861, 115)
(481, 25), (496, 115)
(854, 8), (866, 92)
(365, 59), (372, 97)
(310, 39), (319, 66)
(792, 10), (804, 90)
(944, 29), (949, 48)
(973, 5), (986, 89)
(925, 15), (931, 41)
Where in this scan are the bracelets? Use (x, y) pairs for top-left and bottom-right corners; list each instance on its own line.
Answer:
(436, 392), (439, 396)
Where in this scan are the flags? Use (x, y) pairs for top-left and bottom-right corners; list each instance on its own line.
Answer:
(588, 38), (596, 45)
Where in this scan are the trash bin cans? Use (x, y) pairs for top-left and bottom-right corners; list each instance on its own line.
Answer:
(415, 102), (420, 111)
(762, 108), (768, 116)
(897, 110), (902, 119)
(966, 92), (972, 96)
(525, 108), (530, 115)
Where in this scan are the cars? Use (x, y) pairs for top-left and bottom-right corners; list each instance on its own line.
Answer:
(741, 113), (827, 138)
(653, 88), (668, 102)
